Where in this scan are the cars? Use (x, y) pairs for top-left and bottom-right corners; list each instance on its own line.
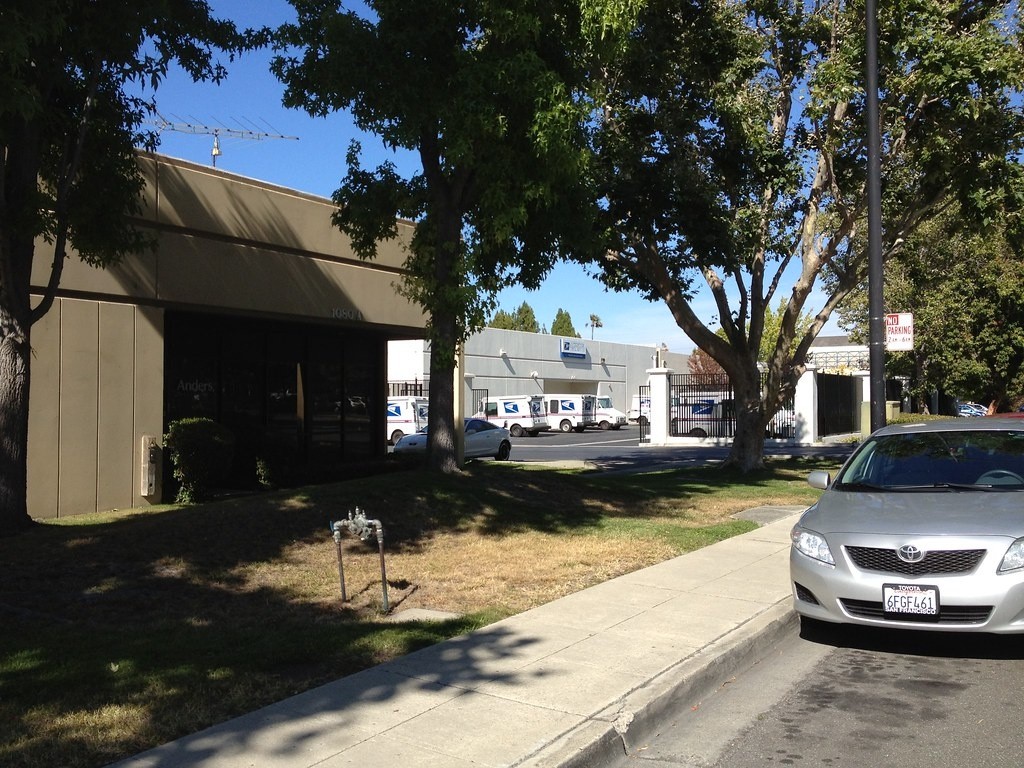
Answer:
(789, 418), (1024, 637)
(270, 389), (367, 409)
(392, 418), (512, 461)
(957, 402), (1024, 419)
(629, 393), (679, 426)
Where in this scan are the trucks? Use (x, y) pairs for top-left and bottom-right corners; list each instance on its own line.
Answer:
(594, 396), (628, 429)
(536, 393), (599, 434)
(677, 392), (797, 438)
(386, 395), (429, 447)
(471, 394), (551, 437)
